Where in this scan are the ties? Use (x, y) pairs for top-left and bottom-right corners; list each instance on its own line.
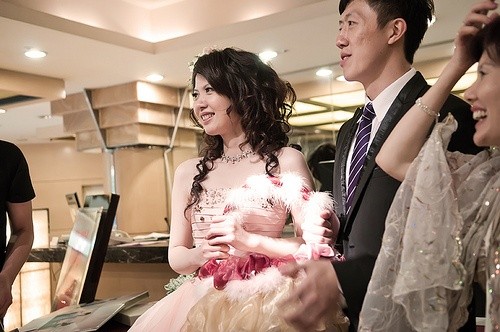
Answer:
(345, 103), (376, 217)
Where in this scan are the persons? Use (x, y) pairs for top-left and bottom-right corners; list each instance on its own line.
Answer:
(273, 0), (489, 332)
(356, 0), (500, 332)
(128, 47), (334, 332)
(0, 139), (36, 332)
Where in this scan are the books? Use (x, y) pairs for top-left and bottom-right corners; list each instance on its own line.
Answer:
(133, 233), (169, 241)
(15, 291), (158, 332)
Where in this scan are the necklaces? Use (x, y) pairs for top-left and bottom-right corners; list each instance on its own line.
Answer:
(220, 152), (254, 165)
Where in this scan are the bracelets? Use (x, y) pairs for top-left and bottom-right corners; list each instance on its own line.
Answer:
(416, 98), (440, 119)
